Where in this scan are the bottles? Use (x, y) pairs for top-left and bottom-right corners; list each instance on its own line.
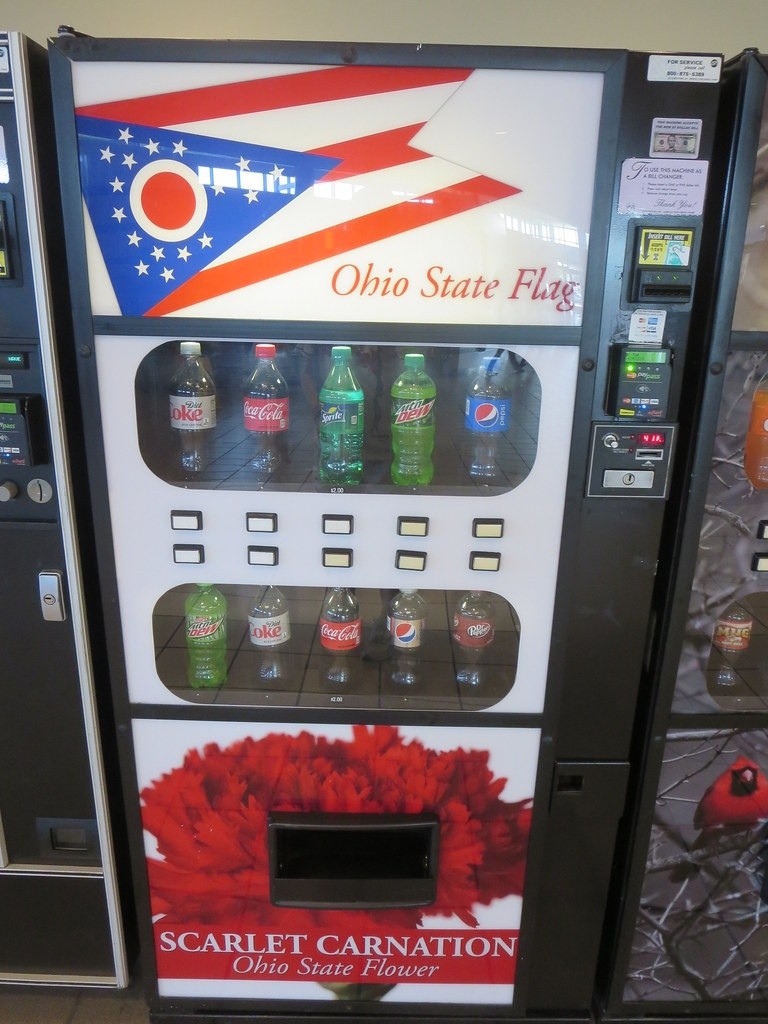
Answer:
(246, 586), (297, 682)
(465, 356), (511, 487)
(186, 583), (229, 688)
(244, 345), (289, 471)
(709, 600), (751, 687)
(387, 588), (427, 685)
(392, 353), (435, 489)
(167, 343), (216, 471)
(454, 590), (494, 686)
(319, 587), (363, 684)
(320, 346), (365, 489)
